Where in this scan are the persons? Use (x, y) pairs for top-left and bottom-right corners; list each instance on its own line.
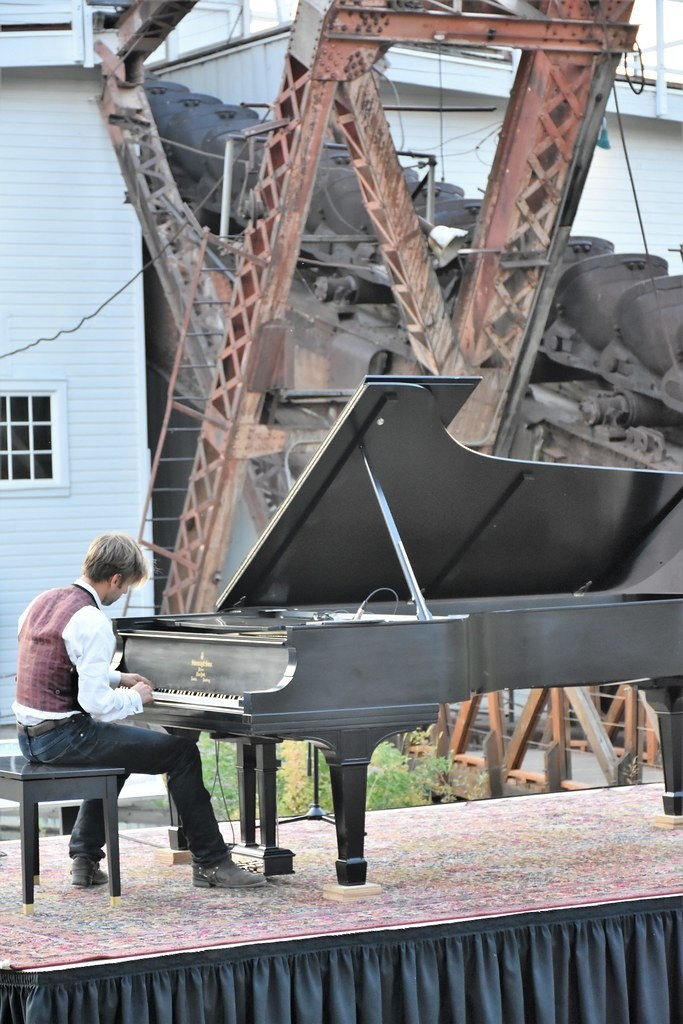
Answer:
(11, 526), (269, 891)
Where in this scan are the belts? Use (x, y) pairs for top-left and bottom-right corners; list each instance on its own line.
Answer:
(16, 713), (86, 738)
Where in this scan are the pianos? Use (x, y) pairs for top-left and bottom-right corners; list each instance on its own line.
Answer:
(117, 373), (683, 887)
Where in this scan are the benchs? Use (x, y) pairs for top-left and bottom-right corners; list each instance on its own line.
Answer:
(0, 756), (125, 916)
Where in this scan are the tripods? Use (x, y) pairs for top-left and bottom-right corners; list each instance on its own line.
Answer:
(253, 739), (367, 836)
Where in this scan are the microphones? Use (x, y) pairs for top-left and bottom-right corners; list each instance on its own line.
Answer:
(353, 600), (368, 619)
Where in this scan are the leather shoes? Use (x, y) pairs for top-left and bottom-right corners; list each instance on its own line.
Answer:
(192, 855), (267, 888)
(69, 855), (108, 885)
